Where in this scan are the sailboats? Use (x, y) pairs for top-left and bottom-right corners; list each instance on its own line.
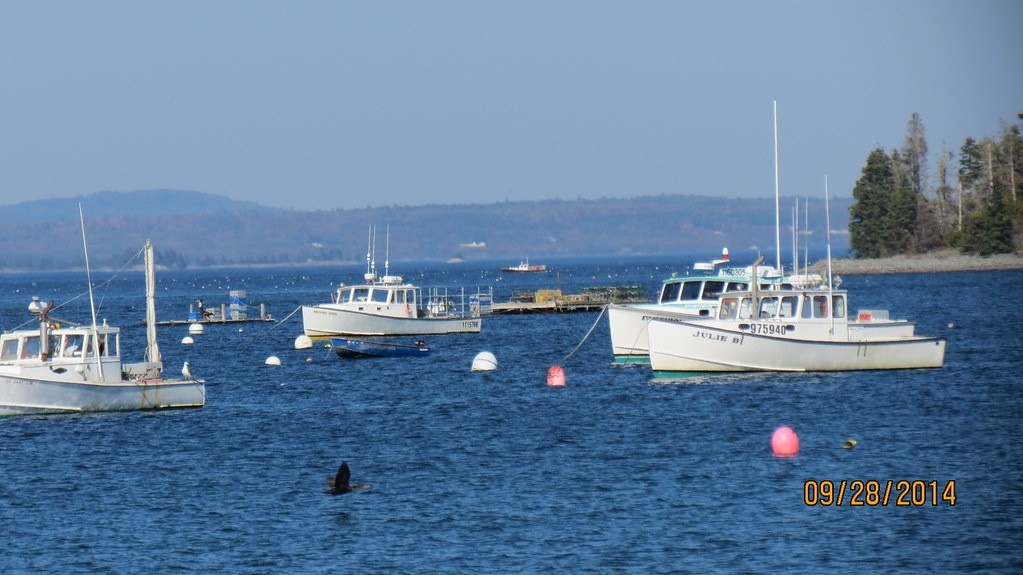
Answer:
(604, 191), (826, 365)
(643, 100), (949, 379)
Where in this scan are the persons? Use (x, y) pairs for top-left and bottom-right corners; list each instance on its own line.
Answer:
(820, 296), (828, 317)
(391, 291), (409, 303)
(427, 297), (457, 317)
(72, 335), (104, 356)
(470, 299), (478, 317)
(198, 298), (206, 320)
(38, 315), (60, 359)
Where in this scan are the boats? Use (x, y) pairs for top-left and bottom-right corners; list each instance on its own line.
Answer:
(1, 202), (206, 418)
(300, 225), (481, 340)
(501, 254), (547, 273)
(329, 337), (431, 361)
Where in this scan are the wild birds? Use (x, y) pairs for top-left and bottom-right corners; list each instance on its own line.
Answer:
(320, 462), (372, 495)
